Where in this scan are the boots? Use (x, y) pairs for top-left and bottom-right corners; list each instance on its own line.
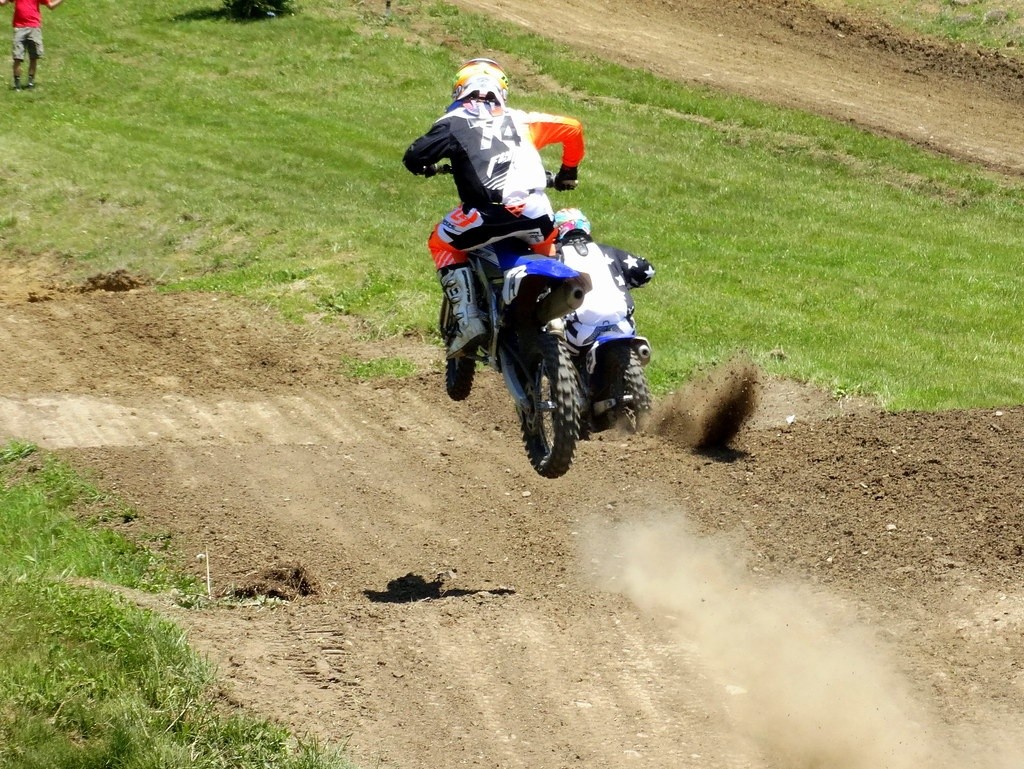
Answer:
(440, 267), (488, 360)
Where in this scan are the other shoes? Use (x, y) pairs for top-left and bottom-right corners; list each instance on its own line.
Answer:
(16, 84), (22, 89)
(26, 82), (35, 88)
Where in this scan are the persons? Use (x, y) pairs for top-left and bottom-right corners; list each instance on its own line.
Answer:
(555, 209), (655, 357)
(0, 0), (63, 92)
(403, 57), (585, 360)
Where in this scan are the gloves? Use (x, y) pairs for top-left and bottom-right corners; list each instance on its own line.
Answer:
(554, 163), (578, 191)
(425, 164), (436, 178)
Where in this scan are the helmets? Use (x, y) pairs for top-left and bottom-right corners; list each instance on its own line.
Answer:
(553, 207), (591, 241)
(451, 58), (509, 106)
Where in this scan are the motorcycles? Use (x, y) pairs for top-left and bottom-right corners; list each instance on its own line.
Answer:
(412, 157), (650, 479)
(584, 315), (658, 433)
